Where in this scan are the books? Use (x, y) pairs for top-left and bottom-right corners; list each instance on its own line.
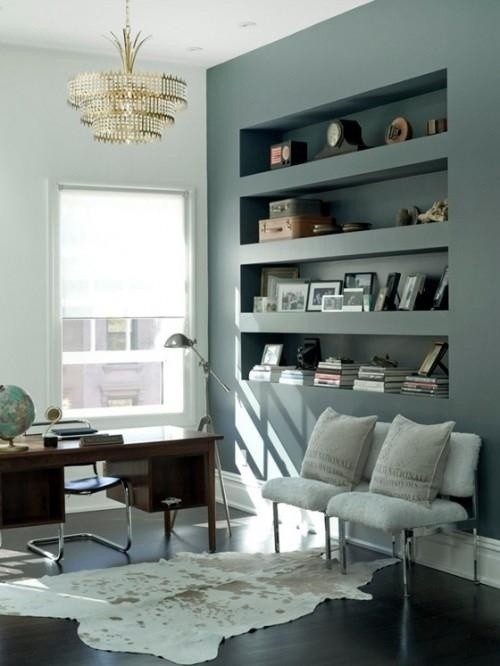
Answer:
(249, 358), (449, 399)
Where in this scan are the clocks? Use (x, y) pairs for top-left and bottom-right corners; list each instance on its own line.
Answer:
(312, 118), (373, 160)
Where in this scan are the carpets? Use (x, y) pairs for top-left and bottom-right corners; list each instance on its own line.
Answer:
(0, 541), (402, 666)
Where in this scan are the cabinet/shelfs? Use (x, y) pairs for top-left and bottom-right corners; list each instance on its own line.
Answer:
(239, 67), (451, 401)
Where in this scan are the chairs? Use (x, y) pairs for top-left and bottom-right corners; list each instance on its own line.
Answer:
(260, 419), (392, 570)
(23, 419), (133, 562)
(327, 431), (482, 597)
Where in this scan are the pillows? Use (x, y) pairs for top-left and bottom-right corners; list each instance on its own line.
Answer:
(368, 412), (457, 509)
(300, 405), (378, 493)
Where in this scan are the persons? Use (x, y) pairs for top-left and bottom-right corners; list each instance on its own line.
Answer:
(313, 291), (322, 305)
(286, 292), (297, 310)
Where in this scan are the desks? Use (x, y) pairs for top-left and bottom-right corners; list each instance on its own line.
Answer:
(0, 424), (225, 552)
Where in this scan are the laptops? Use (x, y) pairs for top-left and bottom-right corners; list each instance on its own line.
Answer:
(51, 427), (98, 436)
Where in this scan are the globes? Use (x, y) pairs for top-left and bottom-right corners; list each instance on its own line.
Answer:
(1, 385), (35, 453)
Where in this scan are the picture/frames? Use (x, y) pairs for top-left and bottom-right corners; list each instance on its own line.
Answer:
(416, 340), (448, 377)
(274, 280), (309, 311)
(305, 278), (343, 312)
(252, 295), (262, 312)
(260, 342), (283, 366)
(374, 287), (388, 311)
(432, 264), (449, 308)
(344, 271), (375, 295)
(260, 266), (299, 297)
(261, 296), (276, 312)
(382, 271), (402, 310)
(398, 272), (422, 310)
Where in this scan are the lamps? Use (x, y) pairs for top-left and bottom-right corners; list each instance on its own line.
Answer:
(63, 0), (189, 146)
(164, 331), (235, 540)
